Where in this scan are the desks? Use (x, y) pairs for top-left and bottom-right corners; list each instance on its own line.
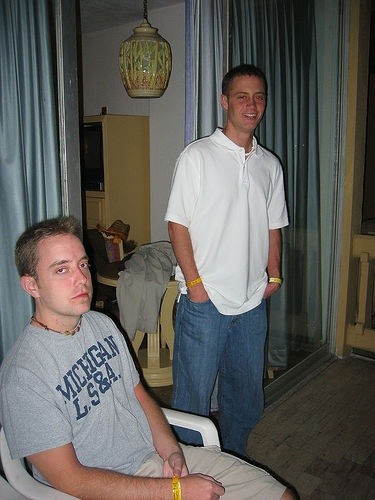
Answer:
(99, 260), (180, 387)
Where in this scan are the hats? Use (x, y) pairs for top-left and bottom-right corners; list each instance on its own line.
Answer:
(96, 220), (130, 241)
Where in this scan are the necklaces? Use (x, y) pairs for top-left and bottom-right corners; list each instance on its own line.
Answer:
(245, 140), (254, 156)
(30, 315), (83, 336)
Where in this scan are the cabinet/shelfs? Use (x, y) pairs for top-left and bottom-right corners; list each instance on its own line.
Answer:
(83, 115), (150, 253)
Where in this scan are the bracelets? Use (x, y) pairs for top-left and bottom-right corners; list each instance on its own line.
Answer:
(185, 277), (202, 287)
(269, 277), (282, 285)
(172, 475), (182, 500)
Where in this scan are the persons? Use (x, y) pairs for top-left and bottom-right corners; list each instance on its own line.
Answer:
(0, 217), (299, 500)
(163, 64), (289, 458)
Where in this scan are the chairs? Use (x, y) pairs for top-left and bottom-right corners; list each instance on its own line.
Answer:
(83, 228), (117, 316)
(0, 406), (222, 500)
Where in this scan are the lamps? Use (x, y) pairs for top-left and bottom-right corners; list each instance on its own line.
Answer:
(119, 0), (172, 98)
